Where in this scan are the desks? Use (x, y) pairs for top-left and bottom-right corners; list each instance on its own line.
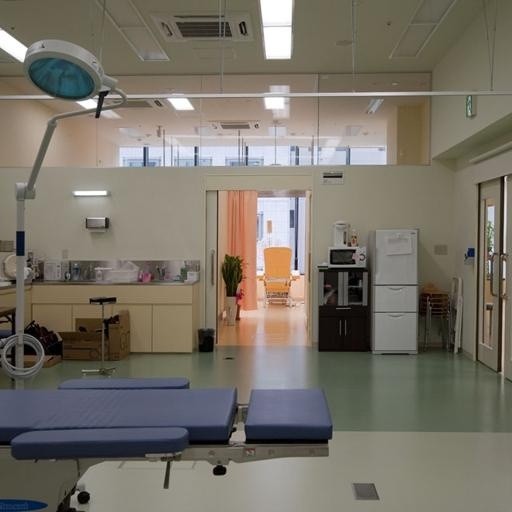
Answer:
(0, 303), (15, 389)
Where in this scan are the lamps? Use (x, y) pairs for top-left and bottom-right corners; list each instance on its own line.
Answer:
(73, 190), (107, 197)
(0, 39), (127, 392)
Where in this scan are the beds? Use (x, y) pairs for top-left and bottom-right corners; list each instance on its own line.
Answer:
(0, 377), (333, 512)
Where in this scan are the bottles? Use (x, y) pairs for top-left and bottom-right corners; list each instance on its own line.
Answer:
(61, 248), (70, 281)
(351, 229), (358, 247)
(154, 264), (160, 281)
(160, 264), (165, 280)
(104, 267), (113, 280)
(94, 266), (103, 281)
(180, 260), (190, 282)
(72, 264), (80, 281)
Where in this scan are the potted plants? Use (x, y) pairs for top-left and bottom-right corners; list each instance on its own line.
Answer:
(222, 254), (242, 327)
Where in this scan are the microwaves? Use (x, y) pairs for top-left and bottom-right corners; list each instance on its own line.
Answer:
(328, 245), (369, 268)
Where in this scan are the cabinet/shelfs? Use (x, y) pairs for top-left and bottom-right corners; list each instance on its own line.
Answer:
(0, 284), (201, 353)
(316, 265), (371, 352)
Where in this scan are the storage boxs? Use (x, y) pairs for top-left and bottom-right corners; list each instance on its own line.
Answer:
(24, 355), (61, 368)
(58, 309), (131, 361)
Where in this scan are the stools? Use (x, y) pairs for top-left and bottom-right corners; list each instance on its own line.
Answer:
(419, 289), (452, 353)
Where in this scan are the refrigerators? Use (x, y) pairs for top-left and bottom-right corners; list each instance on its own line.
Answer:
(371, 228), (419, 355)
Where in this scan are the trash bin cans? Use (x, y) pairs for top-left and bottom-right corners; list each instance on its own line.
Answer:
(198, 328), (215, 352)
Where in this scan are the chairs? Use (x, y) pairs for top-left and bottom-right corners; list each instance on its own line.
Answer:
(258, 247), (300, 306)
